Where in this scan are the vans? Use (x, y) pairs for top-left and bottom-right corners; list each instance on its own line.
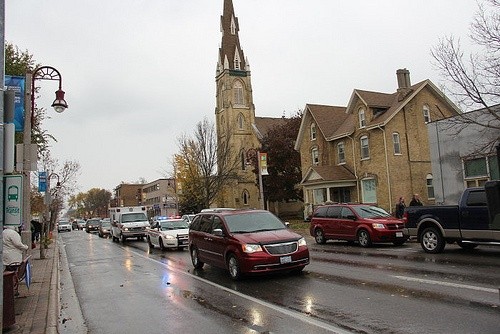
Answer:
(309, 203), (407, 250)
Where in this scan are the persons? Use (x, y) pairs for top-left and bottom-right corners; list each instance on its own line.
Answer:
(396, 196), (407, 219)
(409, 194), (423, 206)
(3, 224), (29, 271)
(30, 220), (47, 242)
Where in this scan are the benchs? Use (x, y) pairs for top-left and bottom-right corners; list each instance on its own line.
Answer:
(3, 255), (33, 305)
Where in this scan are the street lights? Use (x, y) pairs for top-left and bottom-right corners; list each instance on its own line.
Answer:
(20, 66), (69, 252)
(45, 172), (61, 238)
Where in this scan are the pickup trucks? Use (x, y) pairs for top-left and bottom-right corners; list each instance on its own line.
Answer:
(111, 212), (151, 243)
(405, 179), (499, 255)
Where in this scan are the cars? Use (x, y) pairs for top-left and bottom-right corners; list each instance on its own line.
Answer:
(180, 206), (310, 282)
(56, 217), (114, 239)
(145, 213), (190, 251)
